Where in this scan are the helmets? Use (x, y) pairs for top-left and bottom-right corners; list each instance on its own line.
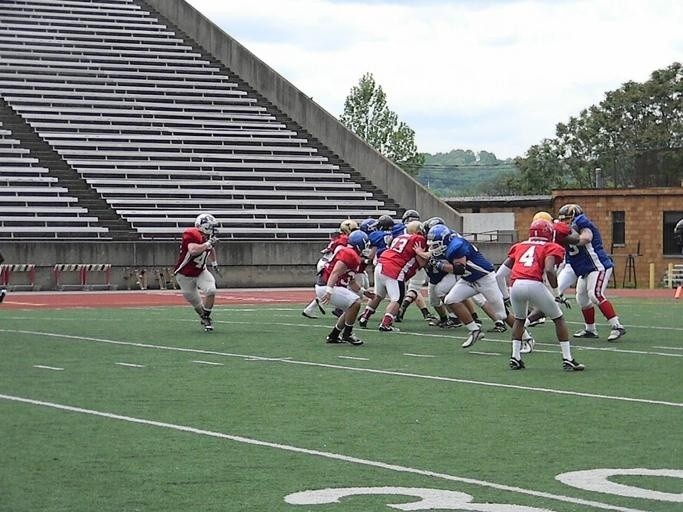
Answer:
(406, 220), (427, 235)
(426, 224), (452, 257)
(348, 229), (369, 253)
(532, 210), (554, 223)
(359, 218), (379, 235)
(528, 218), (554, 242)
(194, 213), (219, 235)
(557, 202), (583, 225)
(378, 215), (395, 230)
(340, 219), (357, 236)
(426, 216), (446, 230)
(402, 209), (420, 224)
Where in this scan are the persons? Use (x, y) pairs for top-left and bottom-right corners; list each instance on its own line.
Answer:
(509, 204), (625, 340)
(302, 210), (536, 354)
(173, 213), (221, 331)
(494, 218), (586, 371)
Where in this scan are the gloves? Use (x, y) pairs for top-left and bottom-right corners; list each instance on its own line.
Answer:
(208, 236), (220, 246)
(563, 299), (572, 310)
(427, 259), (444, 272)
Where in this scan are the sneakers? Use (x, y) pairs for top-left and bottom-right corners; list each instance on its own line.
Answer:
(509, 356), (525, 370)
(379, 322), (400, 332)
(438, 315), (463, 329)
(201, 316), (212, 331)
(520, 337), (535, 354)
(573, 328), (599, 339)
(332, 308), (344, 317)
(562, 358), (585, 370)
(302, 308), (318, 319)
(424, 312), (439, 321)
(316, 297), (326, 315)
(487, 322), (508, 333)
(341, 333), (363, 345)
(326, 334), (346, 344)
(359, 318), (367, 329)
(607, 323), (627, 341)
(461, 328), (485, 349)
(429, 316), (442, 325)
